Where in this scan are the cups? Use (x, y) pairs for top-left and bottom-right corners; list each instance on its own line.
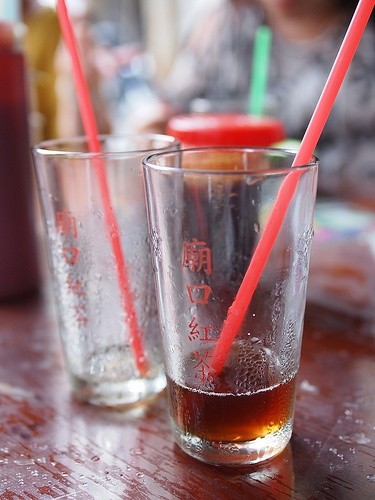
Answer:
(142, 146), (320, 467)
(31, 134), (180, 407)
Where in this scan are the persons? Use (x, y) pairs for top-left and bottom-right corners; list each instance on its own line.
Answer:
(114, 0), (375, 194)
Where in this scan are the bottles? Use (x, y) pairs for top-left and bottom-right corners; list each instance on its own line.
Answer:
(0, 21), (40, 299)
